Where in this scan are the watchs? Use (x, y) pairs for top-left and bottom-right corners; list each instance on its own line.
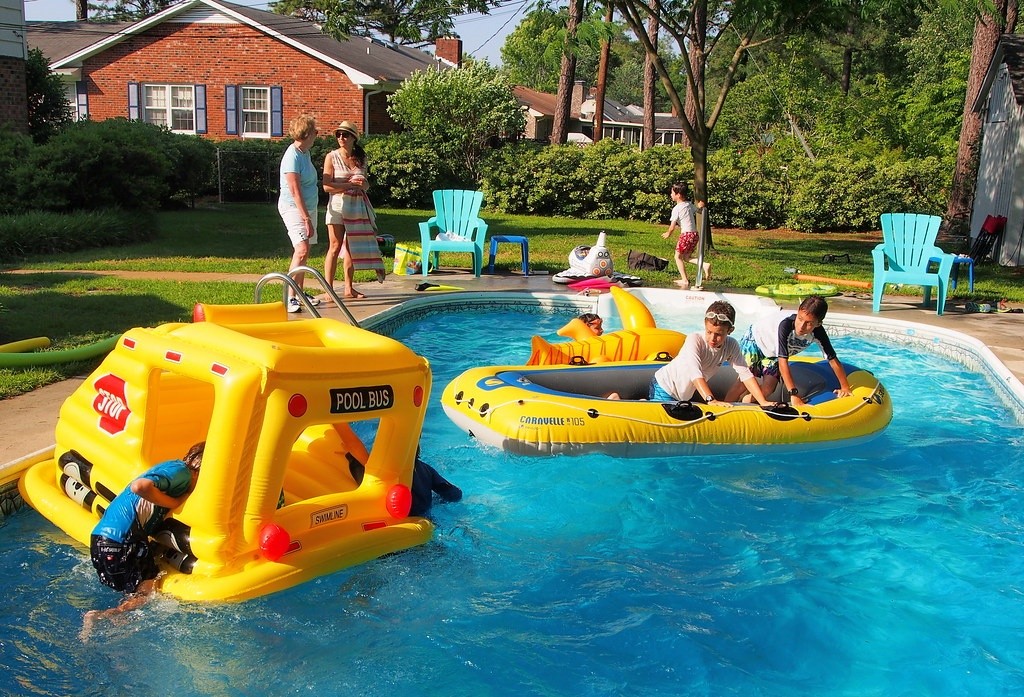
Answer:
(705, 395), (716, 404)
(787, 388), (798, 396)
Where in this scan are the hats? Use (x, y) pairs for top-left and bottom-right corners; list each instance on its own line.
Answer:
(332, 120), (360, 142)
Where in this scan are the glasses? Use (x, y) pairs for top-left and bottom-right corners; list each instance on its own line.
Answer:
(336, 131), (351, 137)
(704, 312), (733, 328)
(590, 317), (603, 324)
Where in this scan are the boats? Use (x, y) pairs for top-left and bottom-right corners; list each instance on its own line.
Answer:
(441, 352), (895, 455)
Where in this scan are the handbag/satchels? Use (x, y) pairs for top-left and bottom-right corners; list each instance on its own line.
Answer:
(393, 241), (434, 277)
(628, 249), (669, 273)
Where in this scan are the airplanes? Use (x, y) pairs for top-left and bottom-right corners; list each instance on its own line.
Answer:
(551, 229), (644, 286)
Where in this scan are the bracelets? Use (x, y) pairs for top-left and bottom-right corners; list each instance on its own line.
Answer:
(303, 216), (311, 221)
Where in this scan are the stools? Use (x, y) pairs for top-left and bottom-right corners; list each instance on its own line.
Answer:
(488, 236), (529, 275)
(924, 254), (974, 292)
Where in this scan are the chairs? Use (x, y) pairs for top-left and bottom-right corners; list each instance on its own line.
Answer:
(872, 213), (954, 313)
(418, 189), (489, 278)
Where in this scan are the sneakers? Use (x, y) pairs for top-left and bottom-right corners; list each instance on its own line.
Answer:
(286, 296), (302, 312)
(988, 301), (1012, 313)
(964, 301), (991, 313)
(295, 291), (320, 306)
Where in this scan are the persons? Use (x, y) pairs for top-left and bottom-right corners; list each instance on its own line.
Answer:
(724, 293), (854, 405)
(661, 182), (711, 286)
(278, 113), (320, 312)
(408, 444), (462, 521)
(579, 313), (603, 336)
(606, 301), (778, 407)
(322, 120), (368, 301)
(81, 441), (205, 644)
